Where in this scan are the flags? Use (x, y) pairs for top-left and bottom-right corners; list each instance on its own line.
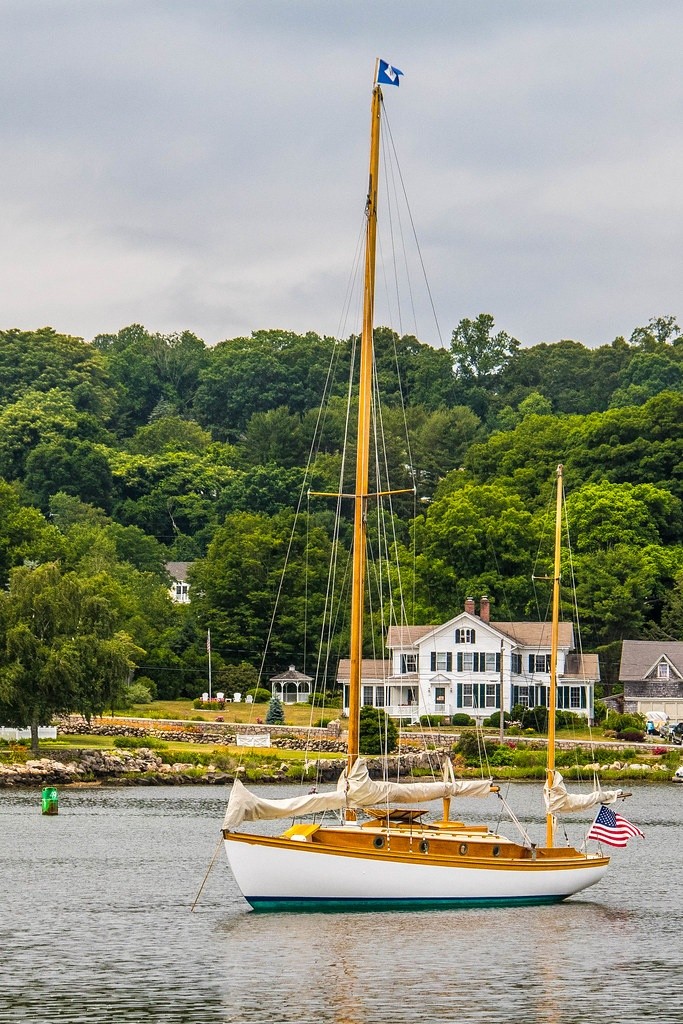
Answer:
(376, 59), (403, 86)
(587, 806), (645, 847)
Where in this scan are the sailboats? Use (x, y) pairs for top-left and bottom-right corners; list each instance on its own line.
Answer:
(218, 52), (612, 915)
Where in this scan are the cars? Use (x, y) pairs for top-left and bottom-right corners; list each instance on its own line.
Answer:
(659, 723), (683, 746)
